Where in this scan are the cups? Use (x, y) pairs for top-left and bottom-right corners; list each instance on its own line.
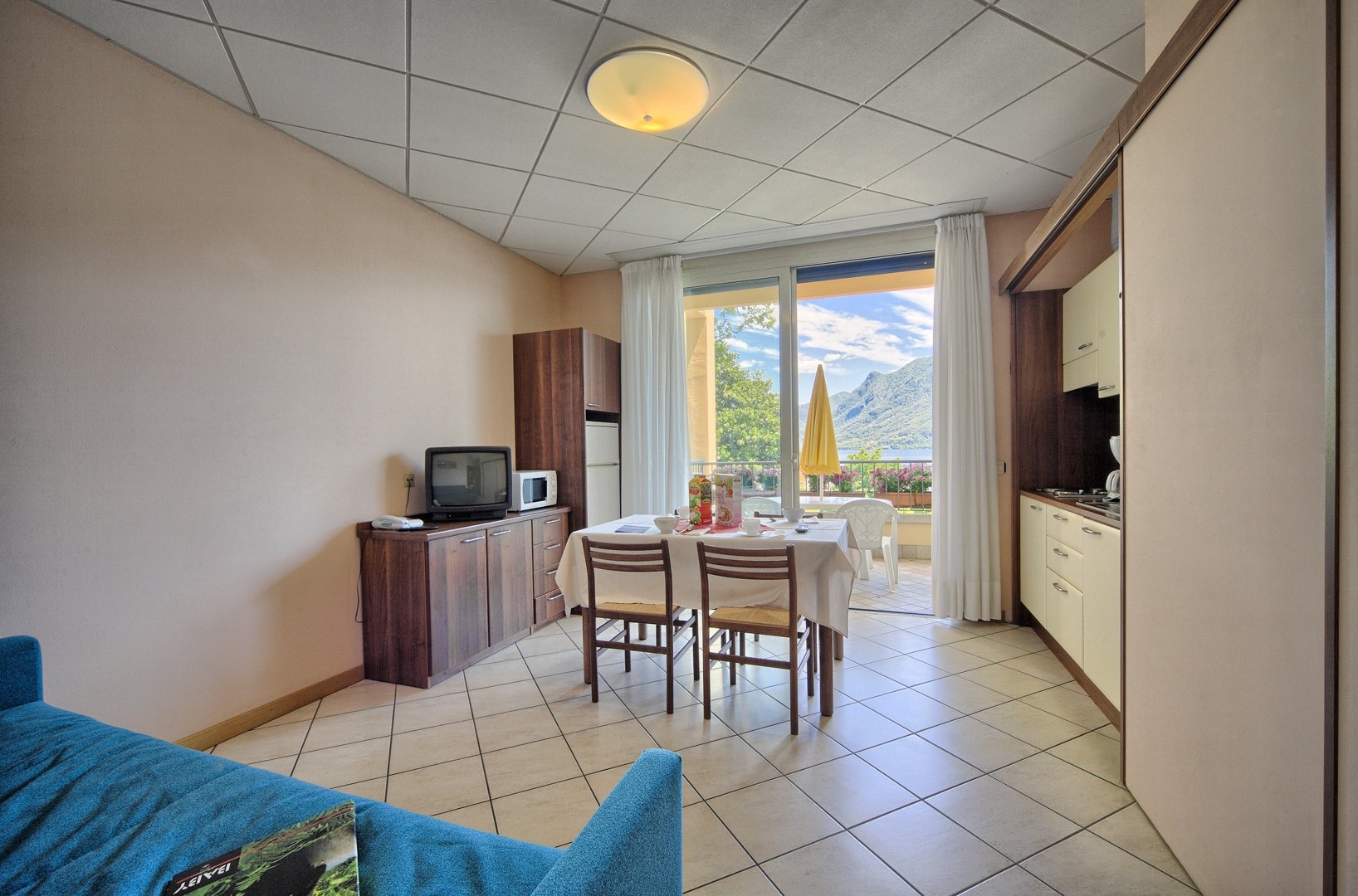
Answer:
(738, 518), (761, 537)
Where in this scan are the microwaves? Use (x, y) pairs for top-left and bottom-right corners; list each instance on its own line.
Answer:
(508, 470), (558, 512)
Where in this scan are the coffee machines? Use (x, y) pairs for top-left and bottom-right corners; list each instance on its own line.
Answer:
(1105, 436), (1120, 500)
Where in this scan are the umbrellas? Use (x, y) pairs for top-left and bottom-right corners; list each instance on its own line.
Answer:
(800, 363), (842, 513)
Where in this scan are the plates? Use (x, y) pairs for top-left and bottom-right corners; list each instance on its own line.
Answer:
(737, 531), (759, 535)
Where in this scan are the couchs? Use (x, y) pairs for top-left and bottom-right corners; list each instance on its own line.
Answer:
(0, 634), (684, 896)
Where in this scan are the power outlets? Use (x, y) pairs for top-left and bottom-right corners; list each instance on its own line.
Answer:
(404, 473), (414, 488)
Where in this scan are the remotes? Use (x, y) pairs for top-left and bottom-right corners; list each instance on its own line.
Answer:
(398, 524), (439, 532)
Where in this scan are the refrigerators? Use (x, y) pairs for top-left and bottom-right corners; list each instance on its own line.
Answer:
(586, 422), (620, 527)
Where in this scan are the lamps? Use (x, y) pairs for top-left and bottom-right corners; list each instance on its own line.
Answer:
(585, 46), (708, 134)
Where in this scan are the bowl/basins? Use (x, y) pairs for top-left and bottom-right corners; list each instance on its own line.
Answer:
(782, 508), (805, 522)
(654, 516), (680, 533)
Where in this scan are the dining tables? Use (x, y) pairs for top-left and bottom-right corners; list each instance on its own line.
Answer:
(554, 514), (863, 717)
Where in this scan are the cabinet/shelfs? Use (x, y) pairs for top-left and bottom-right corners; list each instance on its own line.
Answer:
(1062, 247), (1120, 400)
(356, 505), (575, 691)
(1018, 496), (1123, 733)
(583, 328), (623, 413)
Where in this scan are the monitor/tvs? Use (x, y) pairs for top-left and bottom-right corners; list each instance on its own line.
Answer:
(425, 447), (513, 521)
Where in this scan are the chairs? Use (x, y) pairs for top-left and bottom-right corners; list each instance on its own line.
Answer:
(582, 536), (699, 716)
(834, 499), (901, 592)
(695, 540), (814, 733)
(741, 497), (783, 519)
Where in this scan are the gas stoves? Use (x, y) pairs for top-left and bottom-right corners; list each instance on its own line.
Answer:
(1027, 486), (1107, 499)
